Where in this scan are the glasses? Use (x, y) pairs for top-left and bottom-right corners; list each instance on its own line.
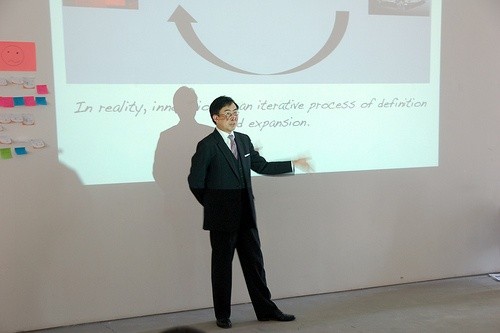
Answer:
(217, 111), (238, 118)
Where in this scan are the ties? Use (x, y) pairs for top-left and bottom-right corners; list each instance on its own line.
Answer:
(228, 135), (238, 159)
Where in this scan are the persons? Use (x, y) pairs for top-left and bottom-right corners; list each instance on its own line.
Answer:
(186, 95), (317, 329)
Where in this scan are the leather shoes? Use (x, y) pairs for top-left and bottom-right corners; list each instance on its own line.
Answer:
(256, 306), (296, 321)
(216, 318), (233, 328)
